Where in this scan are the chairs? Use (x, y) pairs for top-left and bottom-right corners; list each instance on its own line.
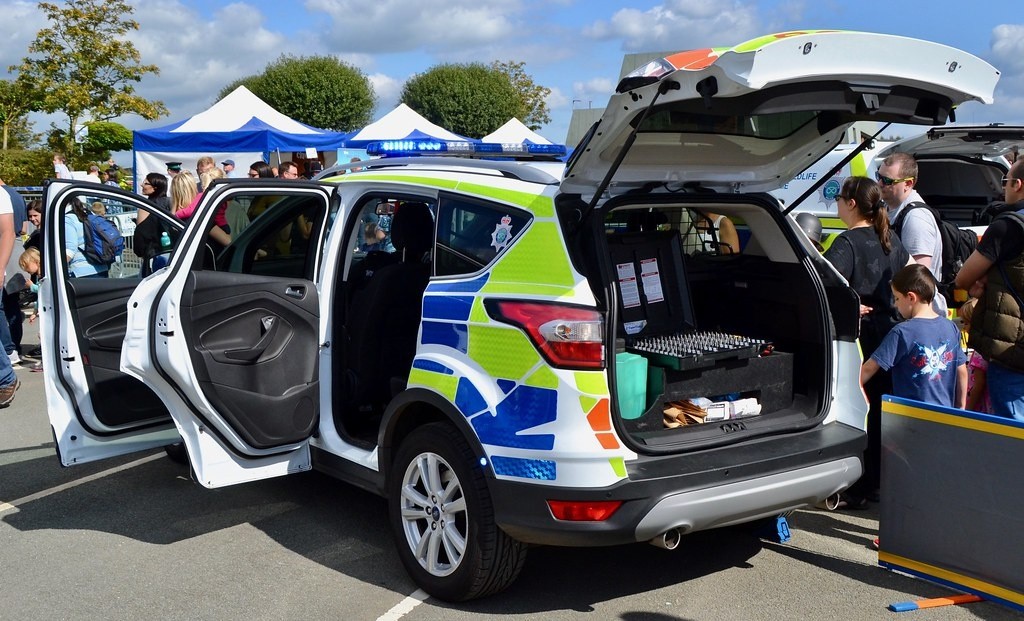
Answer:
(339, 201), (450, 440)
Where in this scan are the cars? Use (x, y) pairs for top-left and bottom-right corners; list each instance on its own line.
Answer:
(878, 122), (1024, 228)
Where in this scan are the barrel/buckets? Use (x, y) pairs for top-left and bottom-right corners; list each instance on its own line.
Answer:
(615, 352), (648, 420)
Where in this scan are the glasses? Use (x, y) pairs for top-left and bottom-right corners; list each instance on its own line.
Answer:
(875, 171), (915, 186)
(834, 194), (845, 201)
(143, 181), (151, 185)
(1001, 175), (1018, 187)
(248, 173), (259, 178)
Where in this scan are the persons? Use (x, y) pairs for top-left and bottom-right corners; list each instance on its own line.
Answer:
(0, 152), (123, 406)
(815, 152), (1024, 549)
(692, 212), (739, 254)
(134, 157), (396, 280)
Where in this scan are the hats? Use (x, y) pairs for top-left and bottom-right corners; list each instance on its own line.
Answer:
(165, 162), (182, 170)
(796, 213), (824, 252)
(90, 165), (103, 175)
(221, 160), (234, 166)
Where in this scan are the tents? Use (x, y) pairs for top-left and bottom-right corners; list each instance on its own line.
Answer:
(133, 84), (346, 211)
(339, 103), (567, 159)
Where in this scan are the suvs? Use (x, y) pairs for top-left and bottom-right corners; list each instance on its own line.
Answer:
(679, 141), (896, 255)
(36, 31), (1001, 602)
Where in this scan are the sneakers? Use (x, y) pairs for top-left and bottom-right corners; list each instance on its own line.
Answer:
(0, 376), (21, 406)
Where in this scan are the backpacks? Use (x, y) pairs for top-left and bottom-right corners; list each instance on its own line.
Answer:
(66, 210), (125, 266)
(889, 202), (979, 309)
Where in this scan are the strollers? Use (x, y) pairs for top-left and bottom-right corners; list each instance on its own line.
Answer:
(2, 273), (38, 348)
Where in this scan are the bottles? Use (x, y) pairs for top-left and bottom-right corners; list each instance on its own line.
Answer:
(161, 232), (171, 246)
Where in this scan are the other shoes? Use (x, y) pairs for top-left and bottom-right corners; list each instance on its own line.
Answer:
(32, 363), (43, 372)
(6, 349), (23, 365)
(25, 347), (42, 358)
